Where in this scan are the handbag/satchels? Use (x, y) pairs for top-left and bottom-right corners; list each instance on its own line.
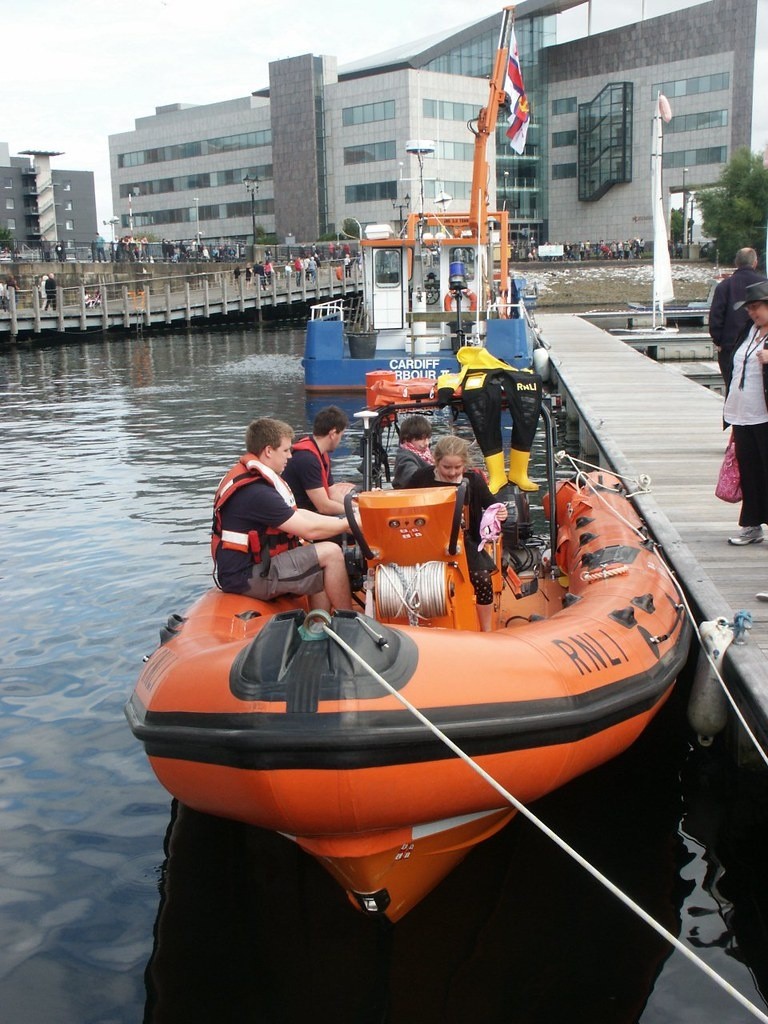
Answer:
(715, 430), (743, 504)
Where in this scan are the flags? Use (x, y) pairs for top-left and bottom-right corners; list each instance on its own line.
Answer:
(505, 27), (530, 155)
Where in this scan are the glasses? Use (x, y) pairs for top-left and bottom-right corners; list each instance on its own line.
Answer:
(743, 301), (764, 312)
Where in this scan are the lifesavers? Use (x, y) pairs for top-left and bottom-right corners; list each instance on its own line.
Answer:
(337, 267), (342, 280)
(444, 288), (477, 312)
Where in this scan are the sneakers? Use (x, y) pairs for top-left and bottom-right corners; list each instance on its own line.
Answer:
(728, 525), (764, 545)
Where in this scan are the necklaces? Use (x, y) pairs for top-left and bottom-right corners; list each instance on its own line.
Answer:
(738, 326), (768, 390)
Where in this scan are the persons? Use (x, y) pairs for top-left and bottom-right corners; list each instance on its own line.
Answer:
(392, 416), (436, 488)
(281, 404), (356, 516)
(114, 236), (149, 262)
(234, 261), (272, 291)
(529, 237), (646, 262)
(41, 273), (56, 311)
(95, 233), (107, 263)
(85, 289), (101, 308)
(265, 247), (273, 261)
(211, 417), (353, 616)
(668, 239), (683, 258)
(710, 248), (768, 546)
(286, 254), (321, 287)
(312, 242), (350, 261)
(162, 239), (245, 264)
(43, 236), (63, 263)
(0, 274), (21, 309)
(411, 434), (508, 632)
(344, 253), (351, 278)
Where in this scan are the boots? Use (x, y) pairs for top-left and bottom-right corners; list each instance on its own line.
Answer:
(508, 447), (540, 492)
(485, 451), (508, 495)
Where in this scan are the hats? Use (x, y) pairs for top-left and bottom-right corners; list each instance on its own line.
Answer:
(733, 280), (768, 311)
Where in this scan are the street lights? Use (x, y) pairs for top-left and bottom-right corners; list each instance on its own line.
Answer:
(193, 198), (203, 244)
(682, 167), (690, 247)
(109, 216), (119, 241)
(242, 174), (262, 262)
(388, 193), (411, 238)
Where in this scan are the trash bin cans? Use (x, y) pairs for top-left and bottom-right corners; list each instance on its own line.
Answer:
(345, 331), (380, 359)
(447, 321), (476, 353)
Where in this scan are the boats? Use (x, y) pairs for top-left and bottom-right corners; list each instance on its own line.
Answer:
(300, 4), (542, 428)
(132, 388), (699, 929)
